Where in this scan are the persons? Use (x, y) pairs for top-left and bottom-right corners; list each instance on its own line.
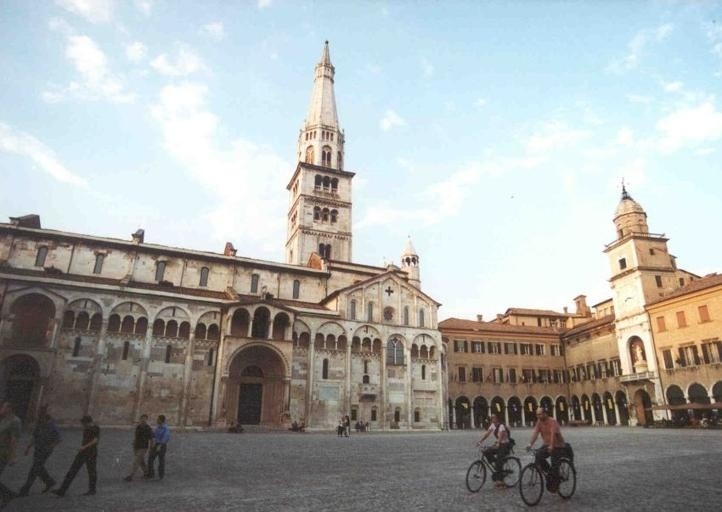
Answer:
(476, 411), (510, 487)
(526, 406), (565, 493)
(123, 414), (154, 482)
(289, 420), (306, 432)
(49, 416), (100, 496)
(228, 420), (244, 433)
(144, 414), (171, 481)
(0, 400), (22, 509)
(335, 415), (370, 437)
(13, 402), (62, 497)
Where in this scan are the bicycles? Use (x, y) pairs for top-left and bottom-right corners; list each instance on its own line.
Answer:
(466, 441), (577, 506)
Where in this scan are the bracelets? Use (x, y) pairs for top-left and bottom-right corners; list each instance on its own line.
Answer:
(528, 444), (532, 448)
